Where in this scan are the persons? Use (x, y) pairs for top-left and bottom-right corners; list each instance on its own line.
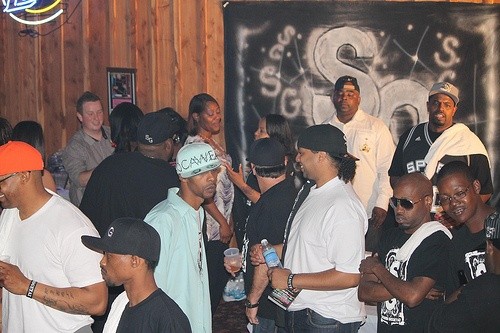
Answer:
(0, 91), (500, 333)
(388, 82), (493, 230)
(111, 73), (129, 97)
(322, 76), (397, 227)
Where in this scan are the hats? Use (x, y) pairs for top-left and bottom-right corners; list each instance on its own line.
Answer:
(0, 140), (44, 175)
(141, 112), (173, 145)
(175, 143), (221, 178)
(298, 124), (359, 161)
(335, 76), (359, 92)
(82, 217), (160, 262)
(430, 82), (459, 105)
(246, 138), (285, 165)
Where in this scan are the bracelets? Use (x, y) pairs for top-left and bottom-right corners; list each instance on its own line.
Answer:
(27, 280), (37, 298)
(287, 273), (296, 289)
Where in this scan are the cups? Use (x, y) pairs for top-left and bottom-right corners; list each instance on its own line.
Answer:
(224, 247), (240, 272)
(0, 255), (10, 287)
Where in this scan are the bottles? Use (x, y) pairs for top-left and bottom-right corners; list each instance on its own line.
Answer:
(261, 239), (282, 270)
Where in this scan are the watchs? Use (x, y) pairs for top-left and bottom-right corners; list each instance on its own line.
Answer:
(245, 299), (259, 308)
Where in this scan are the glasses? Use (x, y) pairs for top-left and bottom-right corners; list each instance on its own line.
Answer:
(389, 194), (430, 210)
(437, 183), (472, 206)
